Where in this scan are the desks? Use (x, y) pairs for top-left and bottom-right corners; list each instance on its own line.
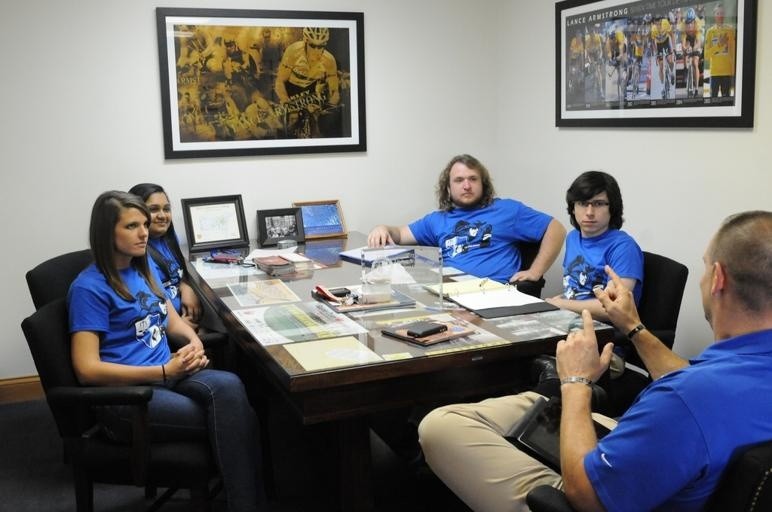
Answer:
(178, 231), (617, 511)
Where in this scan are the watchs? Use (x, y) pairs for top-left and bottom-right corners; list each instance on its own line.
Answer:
(559, 375), (593, 386)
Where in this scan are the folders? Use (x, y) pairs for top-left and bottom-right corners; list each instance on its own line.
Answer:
(338, 244), (415, 266)
(423, 277), (561, 319)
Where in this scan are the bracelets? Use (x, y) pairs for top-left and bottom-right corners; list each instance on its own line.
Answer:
(161, 364), (167, 388)
(623, 324), (646, 338)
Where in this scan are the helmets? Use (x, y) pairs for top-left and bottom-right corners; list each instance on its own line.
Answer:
(685, 8), (695, 24)
(302, 27), (330, 45)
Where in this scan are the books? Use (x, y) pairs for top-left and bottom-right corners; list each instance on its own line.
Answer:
(252, 254), (313, 275)
(381, 316), (476, 347)
(422, 276), (560, 319)
(311, 281), (418, 313)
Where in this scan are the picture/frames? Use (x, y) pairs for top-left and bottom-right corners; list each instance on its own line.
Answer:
(554, 0), (758, 132)
(154, 5), (367, 160)
(181, 194), (250, 254)
(292, 199), (349, 240)
(257, 207), (306, 249)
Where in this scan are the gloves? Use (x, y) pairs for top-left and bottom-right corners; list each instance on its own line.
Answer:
(694, 49), (702, 56)
(654, 54), (660, 66)
(672, 48), (676, 62)
(683, 46), (692, 55)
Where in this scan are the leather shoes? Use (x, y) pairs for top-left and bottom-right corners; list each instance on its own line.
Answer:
(528, 350), (608, 417)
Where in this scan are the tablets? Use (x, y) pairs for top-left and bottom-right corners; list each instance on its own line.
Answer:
(518, 396), (613, 475)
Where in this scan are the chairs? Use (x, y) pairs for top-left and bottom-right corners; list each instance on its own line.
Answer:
(631, 251), (690, 351)
(25, 248), (94, 311)
(523, 441), (772, 512)
(20, 295), (279, 511)
(510, 238), (546, 298)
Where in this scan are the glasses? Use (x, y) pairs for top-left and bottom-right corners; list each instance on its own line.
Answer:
(225, 44), (234, 48)
(262, 34), (271, 39)
(572, 197), (610, 213)
(308, 43), (326, 49)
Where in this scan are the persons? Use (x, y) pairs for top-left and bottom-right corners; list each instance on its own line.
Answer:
(366, 151), (567, 296)
(418, 208), (772, 512)
(173, 23), (349, 144)
(566, 3), (737, 103)
(68, 188), (260, 512)
(127, 182), (202, 370)
(543, 167), (645, 335)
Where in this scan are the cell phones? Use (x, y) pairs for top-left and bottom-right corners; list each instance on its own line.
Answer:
(317, 288), (351, 299)
(407, 323), (447, 338)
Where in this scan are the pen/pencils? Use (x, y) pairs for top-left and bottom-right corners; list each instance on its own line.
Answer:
(479, 276), (488, 289)
(362, 247), (401, 250)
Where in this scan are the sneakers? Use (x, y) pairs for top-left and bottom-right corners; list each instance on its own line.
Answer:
(668, 87), (677, 100)
(660, 87), (666, 99)
(694, 89), (699, 97)
(688, 89), (694, 96)
